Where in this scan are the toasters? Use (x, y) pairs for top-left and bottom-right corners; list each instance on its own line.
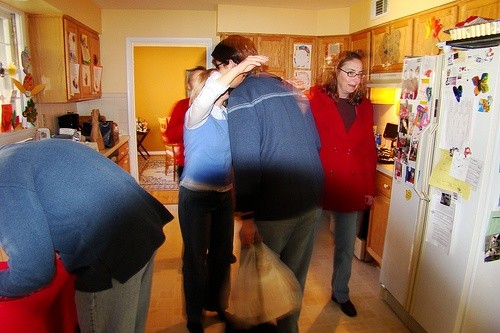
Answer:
(83, 121), (116, 148)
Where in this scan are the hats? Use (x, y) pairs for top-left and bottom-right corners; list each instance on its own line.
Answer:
(225, 242), (302, 330)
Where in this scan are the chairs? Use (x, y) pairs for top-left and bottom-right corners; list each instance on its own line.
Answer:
(157, 116), (179, 181)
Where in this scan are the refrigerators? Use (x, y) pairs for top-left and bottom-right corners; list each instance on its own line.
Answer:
(377, 42), (500, 333)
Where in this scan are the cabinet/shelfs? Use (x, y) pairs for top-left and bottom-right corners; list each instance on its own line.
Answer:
(363, 172), (392, 268)
(27, 14), (102, 104)
(216, 0), (500, 105)
(117, 141), (130, 173)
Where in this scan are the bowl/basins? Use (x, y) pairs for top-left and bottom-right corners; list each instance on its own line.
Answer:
(57, 112), (80, 130)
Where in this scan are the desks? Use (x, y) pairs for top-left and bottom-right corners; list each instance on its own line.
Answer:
(137, 129), (151, 160)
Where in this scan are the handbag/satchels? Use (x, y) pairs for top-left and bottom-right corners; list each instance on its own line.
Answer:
(58, 114), (115, 148)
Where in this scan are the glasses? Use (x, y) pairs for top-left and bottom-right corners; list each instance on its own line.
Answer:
(338, 68), (366, 79)
(215, 61), (228, 70)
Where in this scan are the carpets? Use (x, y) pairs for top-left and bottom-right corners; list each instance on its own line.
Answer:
(138, 159), (180, 190)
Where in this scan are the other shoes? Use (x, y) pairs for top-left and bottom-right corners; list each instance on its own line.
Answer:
(217, 311), (225, 322)
(332, 294), (357, 317)
(187, 315), (203, 333)
(231, 255), (236, 263)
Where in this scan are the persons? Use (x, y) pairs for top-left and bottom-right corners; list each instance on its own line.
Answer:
(164, 34), (325, 333)
(304, 53), (378, 317)
(0, 139), (175, 333)
